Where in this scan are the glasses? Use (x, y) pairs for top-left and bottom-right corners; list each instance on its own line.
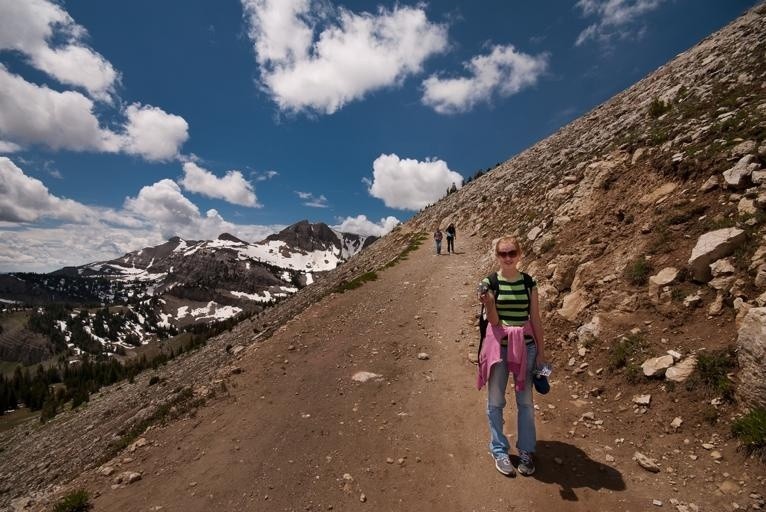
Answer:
(497, 250), (518, 259)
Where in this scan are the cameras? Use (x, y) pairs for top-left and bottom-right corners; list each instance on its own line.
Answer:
(477, 281), (488, 296)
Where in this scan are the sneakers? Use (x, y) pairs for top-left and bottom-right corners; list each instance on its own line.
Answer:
(516, 447), (536, 476)
(491, 452), (515, 476)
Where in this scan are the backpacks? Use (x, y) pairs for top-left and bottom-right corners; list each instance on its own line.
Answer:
(479, 271), (533, 338)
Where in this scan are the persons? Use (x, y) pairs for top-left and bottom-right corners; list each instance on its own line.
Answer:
(434, 227), (443, 255)
(445, 224), (456, 254)
(477, 237), (547, 477)
